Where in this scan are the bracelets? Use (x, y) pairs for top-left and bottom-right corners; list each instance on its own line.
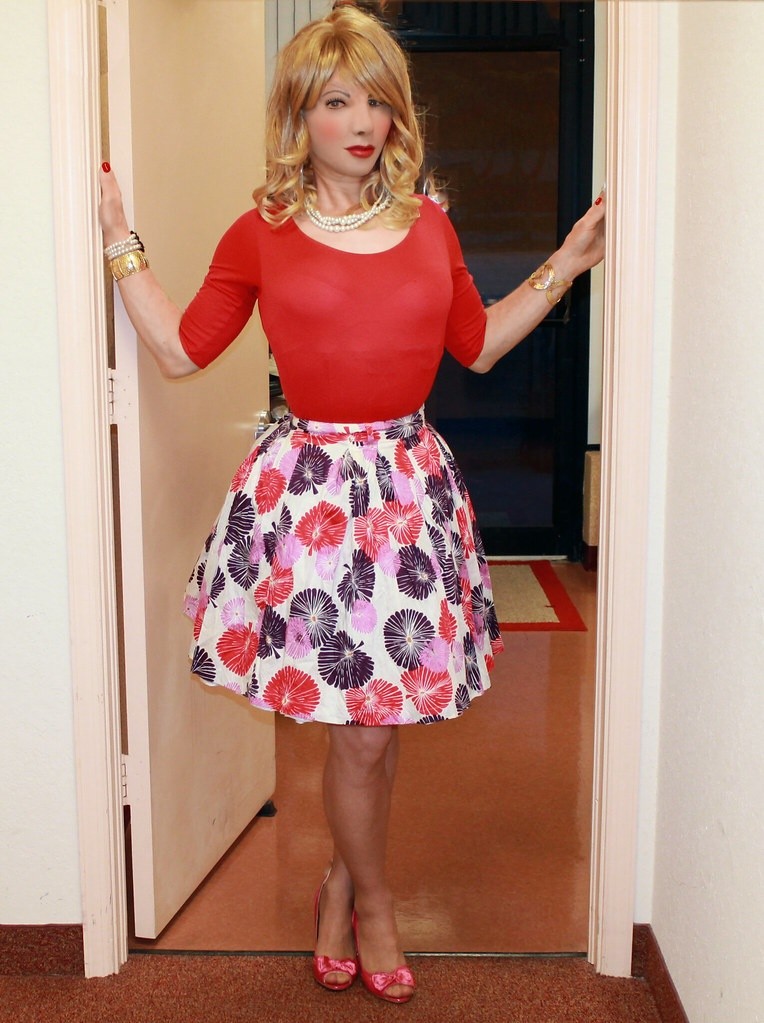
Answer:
(108, 249), (150, 281)
(529, 261), (573, 306)
(103, 228), (146, 259)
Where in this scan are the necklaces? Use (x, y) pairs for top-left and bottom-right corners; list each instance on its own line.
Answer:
(303, 187), (394, 234)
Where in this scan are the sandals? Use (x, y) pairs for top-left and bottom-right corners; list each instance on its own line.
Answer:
(312, 889), (415, 1004)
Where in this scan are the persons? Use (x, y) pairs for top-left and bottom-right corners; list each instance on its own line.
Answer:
(93, 5), (613, 997)
